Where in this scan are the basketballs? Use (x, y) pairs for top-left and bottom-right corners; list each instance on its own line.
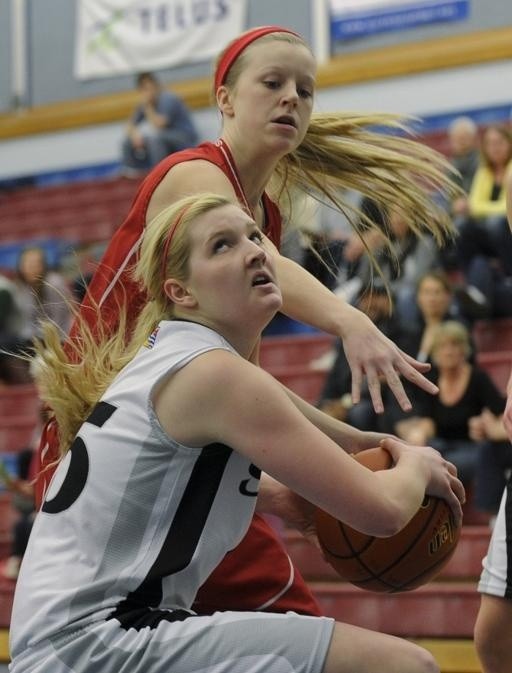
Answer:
(314, 448), (462, 591)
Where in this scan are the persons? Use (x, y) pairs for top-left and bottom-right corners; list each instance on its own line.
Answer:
(472, 370), (511, 673)
(301, 117), (512, 511)
(29, 24), (446, 621)
(120, 69), (197, 178)
(5, 191), (469, 673)
(0, 245), (77, 581)
